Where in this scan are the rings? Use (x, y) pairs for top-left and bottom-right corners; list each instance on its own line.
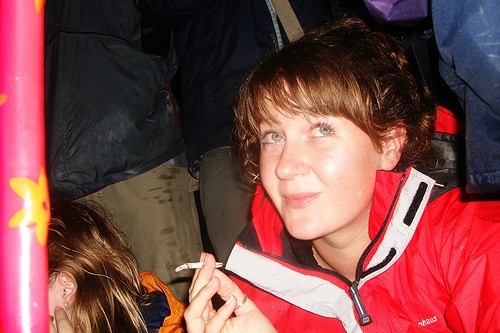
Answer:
(232, 293), (248, 312)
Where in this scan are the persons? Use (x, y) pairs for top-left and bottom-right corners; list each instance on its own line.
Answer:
(49, 196), (189, 333)
(176, 0), (367, 273)
(43, 0), (204, 304)
(183, 19), (500, 333)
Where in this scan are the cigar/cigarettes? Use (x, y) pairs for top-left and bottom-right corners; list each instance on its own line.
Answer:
(174, 261), (225, 275)
